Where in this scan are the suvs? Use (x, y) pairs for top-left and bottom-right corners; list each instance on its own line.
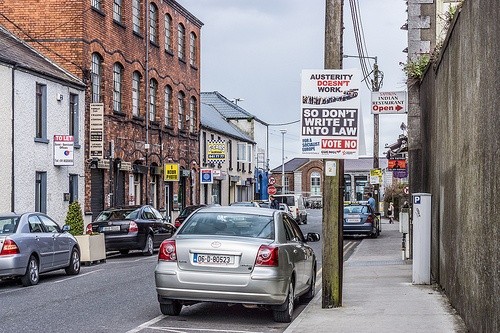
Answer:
(269, 194), (310, 225)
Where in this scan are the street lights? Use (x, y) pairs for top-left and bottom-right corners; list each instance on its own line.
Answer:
(342, 55), (379, 213)
(280, 129), (287, 194)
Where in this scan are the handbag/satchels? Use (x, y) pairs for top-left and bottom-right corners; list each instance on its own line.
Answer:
(387, 211), (392, 215)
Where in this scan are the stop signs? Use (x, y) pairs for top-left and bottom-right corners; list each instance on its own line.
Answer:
(267, 185), (277, 195)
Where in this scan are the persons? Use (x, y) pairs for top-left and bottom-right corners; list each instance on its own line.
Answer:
(311, 201), (321, 209)
(270, 196), (279, 210)
(367, 193), (375, 210)
(305, 201), (309, 208)
(388, 202), (395, 224)
(402, 201), (409, 208)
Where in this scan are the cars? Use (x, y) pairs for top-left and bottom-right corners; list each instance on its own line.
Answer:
(344, 204), (381, 239)
(0, 211), (81, 287)
(154, 204), (321, 323)
(228, 200), (293, 228)
(85, 204), (178, 256)
(174, 204), (227, 233)
(301, 191), (323, 209)
